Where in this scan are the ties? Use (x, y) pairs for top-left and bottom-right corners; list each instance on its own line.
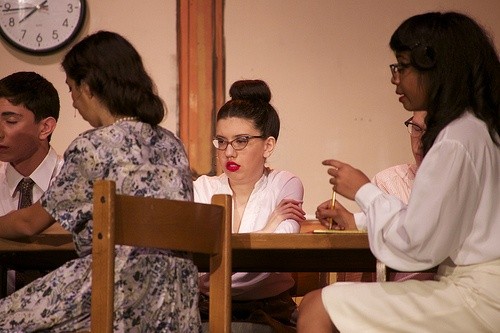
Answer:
(15, 177), (39, 291)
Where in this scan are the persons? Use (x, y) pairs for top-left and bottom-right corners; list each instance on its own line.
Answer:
(192, 81), (306, 332)
(0, 72), (65, 298)
(297, 11), (500, 332)
(0, 31), (202, 333)
(371, 111), (434, 280)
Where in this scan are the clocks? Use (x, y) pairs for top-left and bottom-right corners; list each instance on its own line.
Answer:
(0, 0), (86, 56)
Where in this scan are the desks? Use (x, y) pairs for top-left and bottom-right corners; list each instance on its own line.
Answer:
(0, 232), (387, 300)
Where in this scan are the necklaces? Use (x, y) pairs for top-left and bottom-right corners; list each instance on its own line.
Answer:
(115, 117), (136, 122)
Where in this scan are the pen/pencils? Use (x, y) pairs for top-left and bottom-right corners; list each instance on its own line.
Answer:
(328, 190), (336, 230)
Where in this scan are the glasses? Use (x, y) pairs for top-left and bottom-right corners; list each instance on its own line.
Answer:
(213, 135), (265, 151)
(390, 63), (411, 80)
(404, 116), (425, 137)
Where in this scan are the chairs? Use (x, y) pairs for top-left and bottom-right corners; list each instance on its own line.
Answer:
(90, 180), (232, 333)
